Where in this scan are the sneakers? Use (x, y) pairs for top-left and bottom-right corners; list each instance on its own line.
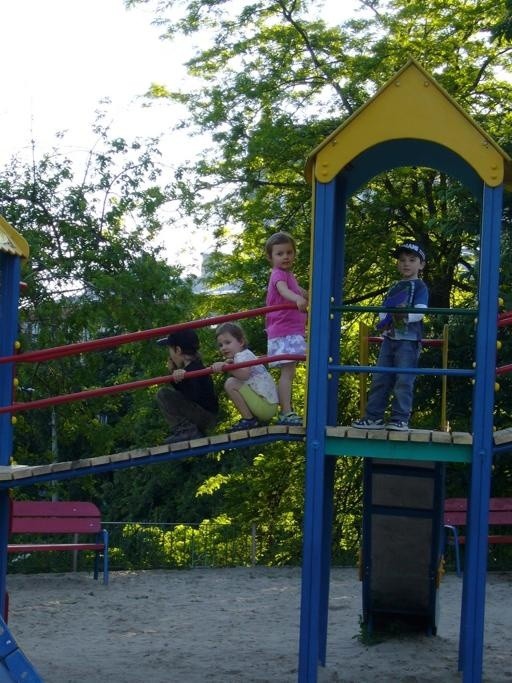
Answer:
(163, 422), (204, 444)
(386, 420), (409, 430)
(277, 411), (304, 425)
(351, 417), (385, 428)
(227, 416), (261, 432)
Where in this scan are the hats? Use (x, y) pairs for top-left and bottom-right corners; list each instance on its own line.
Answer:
(156, 328), (200, 353)
(392, 239), (426, 260)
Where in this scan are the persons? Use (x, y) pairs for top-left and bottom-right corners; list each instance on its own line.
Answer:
(155, 327), (219, 445)
(350, 241), (431, 433)
(262, 228), (310, 427)
(211, 320), (279, 433)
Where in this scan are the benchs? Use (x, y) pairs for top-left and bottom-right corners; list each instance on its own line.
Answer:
(7, 500), (110, 586)
(443, 497), (512, 576)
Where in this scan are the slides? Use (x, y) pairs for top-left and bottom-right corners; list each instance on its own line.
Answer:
(358, 456), (445, 639)
